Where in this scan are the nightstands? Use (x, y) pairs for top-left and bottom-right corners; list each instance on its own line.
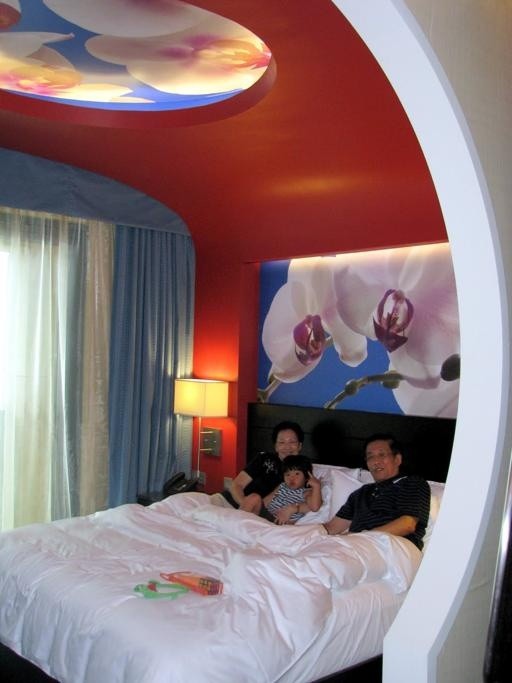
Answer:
(136, 491), (166, 506)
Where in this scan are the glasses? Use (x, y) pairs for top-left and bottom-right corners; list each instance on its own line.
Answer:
(278, 439), (298, 446)
(363, 449), (395, 460)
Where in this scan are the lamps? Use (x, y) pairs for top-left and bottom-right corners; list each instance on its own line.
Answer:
(173, 378), (229, 478)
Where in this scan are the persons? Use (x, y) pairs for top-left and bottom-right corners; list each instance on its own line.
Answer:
(323, 431), (431, 551)
(239, 454), (323, 526)
(210, 420), (313, 525)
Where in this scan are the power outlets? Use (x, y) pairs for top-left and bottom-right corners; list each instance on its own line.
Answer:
(194, 471), (206, 485)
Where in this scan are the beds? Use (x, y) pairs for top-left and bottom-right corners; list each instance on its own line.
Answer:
(1, 403), (456, 683)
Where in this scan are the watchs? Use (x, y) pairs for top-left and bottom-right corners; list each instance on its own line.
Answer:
(295, 502), (300, 513)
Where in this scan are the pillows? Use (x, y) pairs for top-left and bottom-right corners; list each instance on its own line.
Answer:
(295, 463), (361, 524)
(329, 468), (364, 519)
(359, 469), (445, 552)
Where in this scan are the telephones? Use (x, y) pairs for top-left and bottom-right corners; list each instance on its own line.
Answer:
(162, 472), (196, 495)
